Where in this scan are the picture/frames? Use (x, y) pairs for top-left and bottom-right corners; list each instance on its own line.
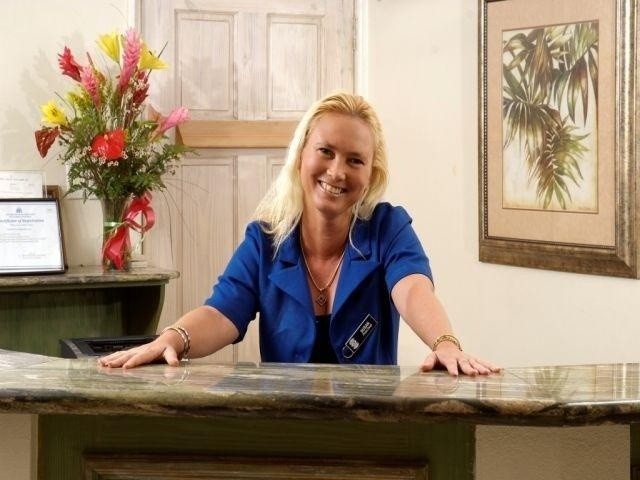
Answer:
(477, 0), (638, 281)
(0, 197), (69, 276)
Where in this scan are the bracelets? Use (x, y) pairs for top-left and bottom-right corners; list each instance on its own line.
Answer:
(161, 325), (191, 360)
(431, 334), (462, 351)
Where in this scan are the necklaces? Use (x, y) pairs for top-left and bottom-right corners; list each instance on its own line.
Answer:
(299, 223), (346, 307)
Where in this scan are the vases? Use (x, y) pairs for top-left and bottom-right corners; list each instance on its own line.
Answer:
(99, 196), (136, 271)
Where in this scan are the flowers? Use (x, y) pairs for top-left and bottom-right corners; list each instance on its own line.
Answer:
(31, 5), (203, 270)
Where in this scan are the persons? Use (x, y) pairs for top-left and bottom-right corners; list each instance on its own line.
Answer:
(97, 94), (501, 378)
